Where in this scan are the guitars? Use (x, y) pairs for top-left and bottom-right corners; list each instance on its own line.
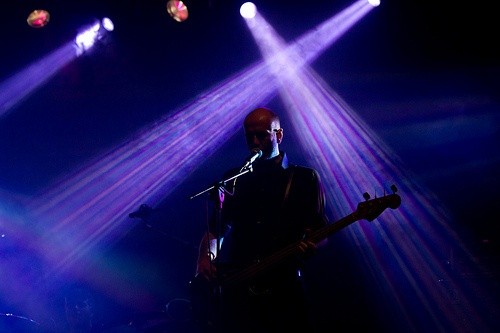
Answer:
(174, 193), (402, 333)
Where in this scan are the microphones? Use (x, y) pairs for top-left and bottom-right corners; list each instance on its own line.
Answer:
(243, 147), (262, 168)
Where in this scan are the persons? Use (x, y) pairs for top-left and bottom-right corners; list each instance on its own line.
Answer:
(196, 107), (328, 333)
